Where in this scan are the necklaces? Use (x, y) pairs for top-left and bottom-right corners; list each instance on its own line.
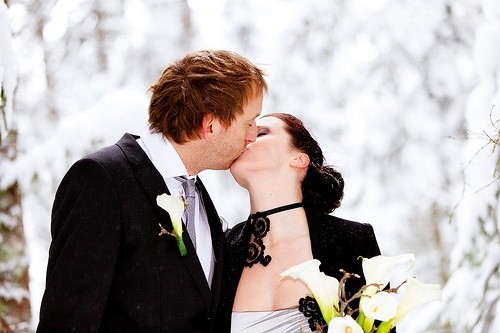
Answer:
(243, 202), (303, 268)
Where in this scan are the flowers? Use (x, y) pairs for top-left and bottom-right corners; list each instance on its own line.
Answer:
(157, 193), (188, 255)
(278, 253), (431, 333)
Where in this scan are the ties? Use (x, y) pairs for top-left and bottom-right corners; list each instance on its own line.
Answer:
(173, 176), (197, 251)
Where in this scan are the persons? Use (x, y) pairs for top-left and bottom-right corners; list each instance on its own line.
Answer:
(36, 49), (268, 333)
(216, 114), (397, 332)
